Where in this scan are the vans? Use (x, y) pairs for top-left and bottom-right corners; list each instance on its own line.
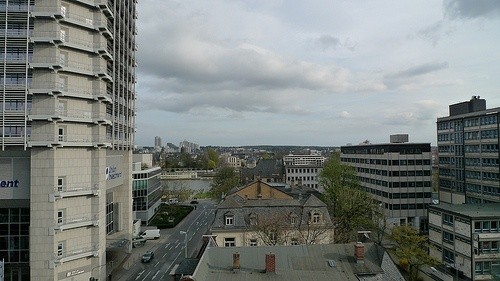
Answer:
(168, 198), (179, 204)
(140, 229), (160, 240)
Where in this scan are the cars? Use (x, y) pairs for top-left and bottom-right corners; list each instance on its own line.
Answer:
(190, 201), (199, 204)
(142, 250), (154, 263)
(132, 236), (146, 248)
